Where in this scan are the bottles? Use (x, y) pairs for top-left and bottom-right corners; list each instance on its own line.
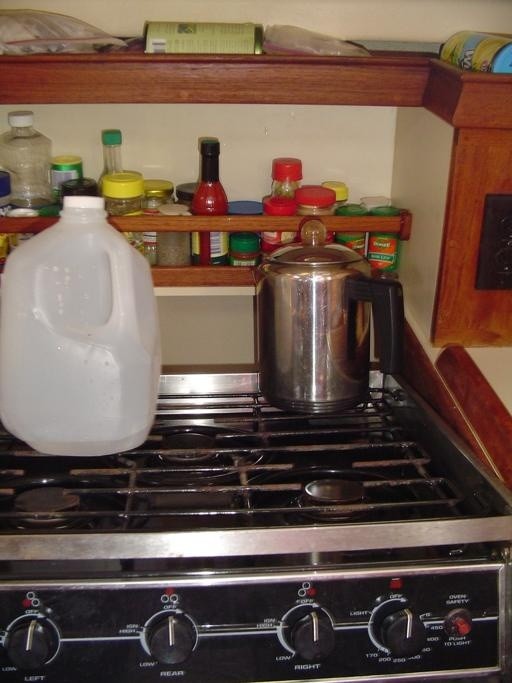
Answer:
(0, 111), (53, 212)
(154, 203), (191, 264)
(98, 129), (127, 195)
(270, 157), (305, 201)
(192, 139), (229, 267)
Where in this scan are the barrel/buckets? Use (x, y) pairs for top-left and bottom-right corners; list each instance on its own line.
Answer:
(0, 195), (160, 457)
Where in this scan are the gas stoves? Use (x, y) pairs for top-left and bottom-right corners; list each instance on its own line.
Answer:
(0, 364), (511, 682)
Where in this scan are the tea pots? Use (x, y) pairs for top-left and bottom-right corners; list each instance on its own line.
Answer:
(253, 221), (404, 413)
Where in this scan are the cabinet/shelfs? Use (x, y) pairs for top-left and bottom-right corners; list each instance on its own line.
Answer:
(0, 30), (430, 300)
